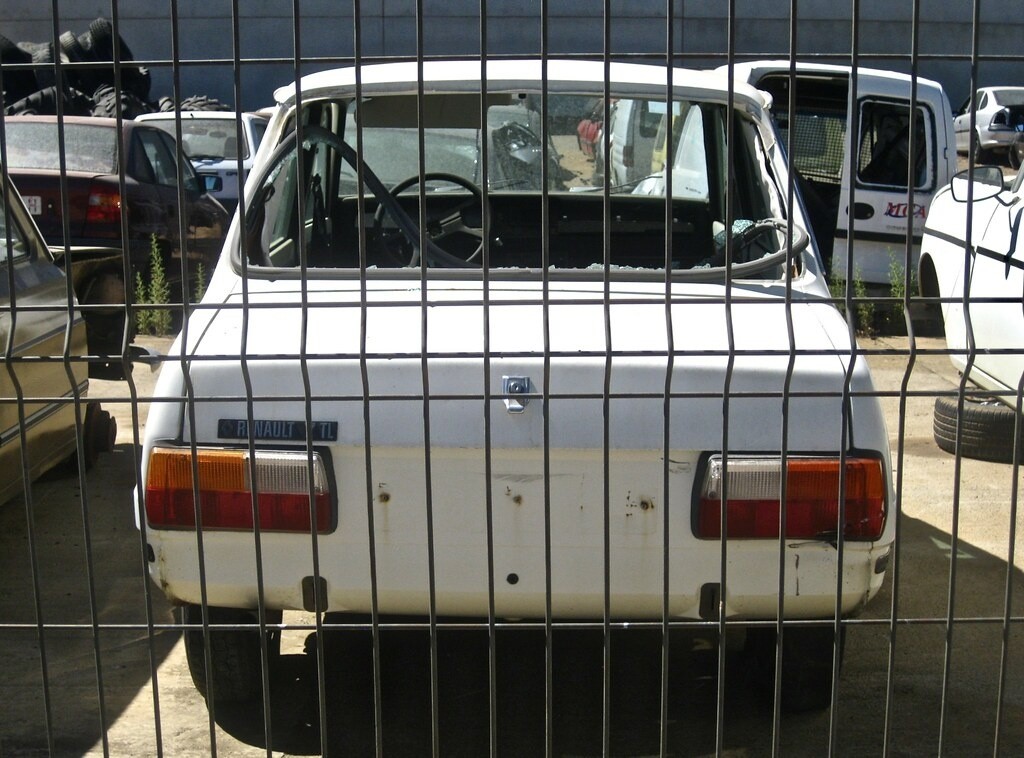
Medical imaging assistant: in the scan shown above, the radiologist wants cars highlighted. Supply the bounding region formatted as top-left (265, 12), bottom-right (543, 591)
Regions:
top-left (135, 54), bottom-right (898, 717)
top-left (595, 99), bottom-right (666, 193)
top-left (311, 116), bottom-right (563, 194)
top-left (3, 115), bottom-right (232, 300)
top-left (132, 110), bottom-right (270, 218)
top-left (576, 97), bottom-right (618, 159)
top-left (650, 102), bottom-right (846, 185)
top-left (954, 86), bottom-right (1024, 163)
top-left (919, 154), bottom-right (1023, 417)
top-left (487, 105), bottom-right (556, 159)
top-left (0, 168), bottom-right (116, 507)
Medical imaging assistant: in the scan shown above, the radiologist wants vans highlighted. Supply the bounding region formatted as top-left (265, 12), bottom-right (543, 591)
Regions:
top-left (631, 60), bottom-right (956, 334)
